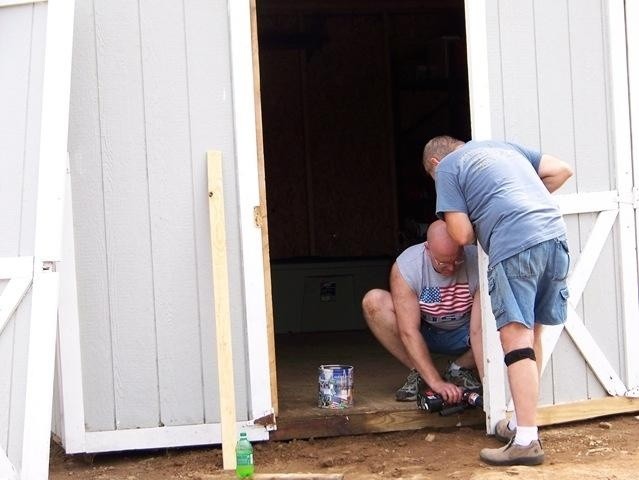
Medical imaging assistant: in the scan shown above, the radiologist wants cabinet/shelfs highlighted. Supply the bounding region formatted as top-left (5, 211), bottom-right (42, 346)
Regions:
top-left (235, 431), bottom-right (255, 478)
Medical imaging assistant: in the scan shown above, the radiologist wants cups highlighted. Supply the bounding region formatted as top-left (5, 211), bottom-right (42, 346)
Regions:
top-left (431, 252), bottom-right (465, 268)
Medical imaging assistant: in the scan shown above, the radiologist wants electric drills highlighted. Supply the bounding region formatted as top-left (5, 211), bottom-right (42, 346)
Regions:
top-left (417, 386), bottom-right (483, 416)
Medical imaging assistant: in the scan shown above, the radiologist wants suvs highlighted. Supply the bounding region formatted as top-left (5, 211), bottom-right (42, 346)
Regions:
top-left (318, 365), bottom-right (354, 408)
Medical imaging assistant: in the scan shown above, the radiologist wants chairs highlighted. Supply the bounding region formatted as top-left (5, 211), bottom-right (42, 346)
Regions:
top-left (394, 369), bottom-right (419, 401)
top-left (439, 360), bottom-right (482, 389)
top-left (494, 418), bottom-right (518, 442)
top-left (480, 435), bottom-right (545, 465)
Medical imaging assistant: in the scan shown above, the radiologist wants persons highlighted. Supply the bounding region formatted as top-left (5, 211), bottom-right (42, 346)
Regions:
top-left (362, 219), bottom-right (485, 406)
top-left (421, 134), bottom-right (577, 468)
